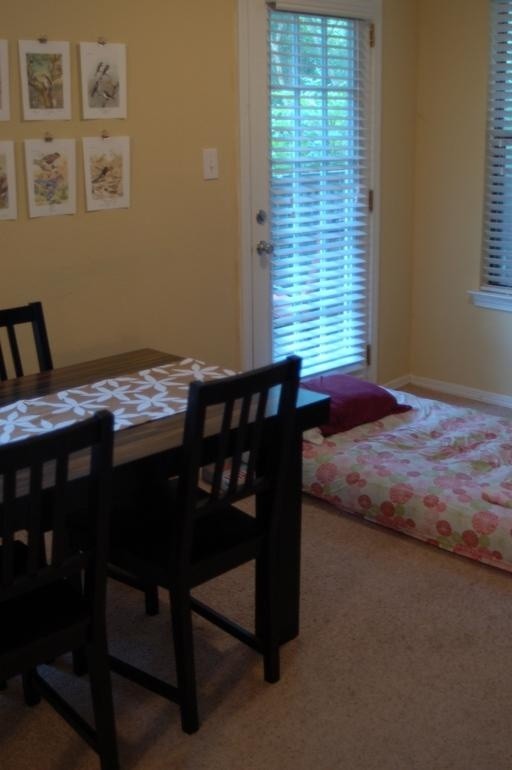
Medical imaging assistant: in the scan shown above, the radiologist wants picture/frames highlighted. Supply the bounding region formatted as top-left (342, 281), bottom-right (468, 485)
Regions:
top-left (0, 38), bottom-right (130, 221)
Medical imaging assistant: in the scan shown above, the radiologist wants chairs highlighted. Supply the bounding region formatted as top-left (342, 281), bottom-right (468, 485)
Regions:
top-left (0, 411), bottom-right (123, 768)
top-left (84, 354), bottom-right (301, 735)
top-left (0, 295), bottom-right (55, 381)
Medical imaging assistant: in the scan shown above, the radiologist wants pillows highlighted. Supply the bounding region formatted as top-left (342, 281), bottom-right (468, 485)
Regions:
top-left (297, 371), bottom-right (412, 446)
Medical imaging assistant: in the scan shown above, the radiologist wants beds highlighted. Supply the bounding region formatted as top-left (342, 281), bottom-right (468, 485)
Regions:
top-left (238, 376), bottom-right (512, 575)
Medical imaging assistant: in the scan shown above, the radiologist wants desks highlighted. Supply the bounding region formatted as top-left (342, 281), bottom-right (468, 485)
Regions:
top-left (1, 346), bottom-right (332, 642)
